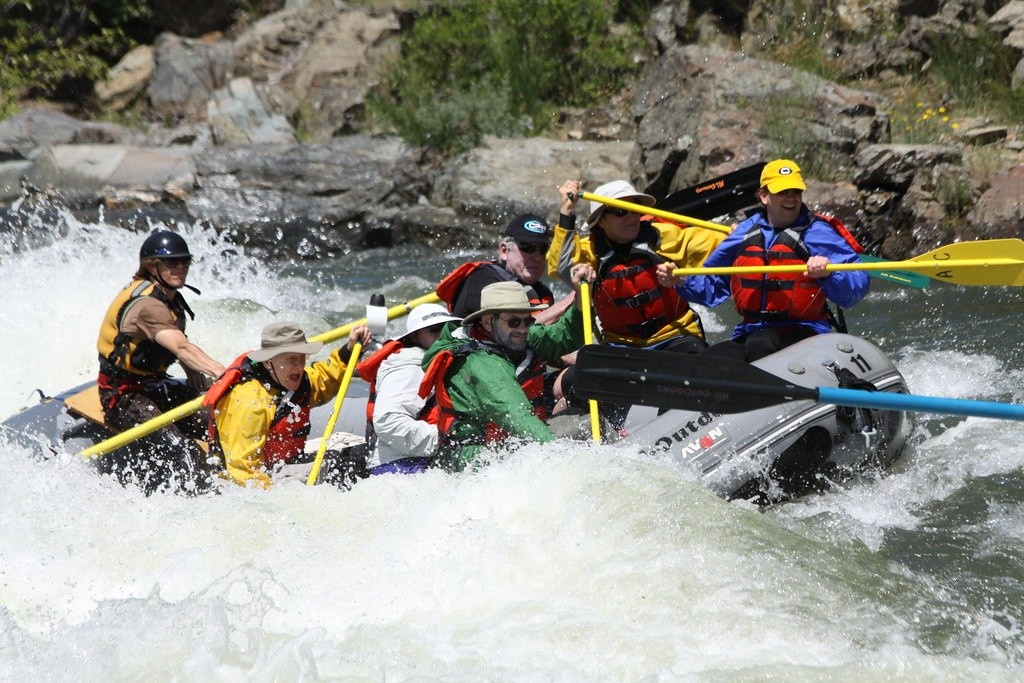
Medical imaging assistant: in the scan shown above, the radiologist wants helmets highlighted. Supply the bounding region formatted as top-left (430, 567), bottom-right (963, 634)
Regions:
top-left (139, 231), bottom-right (192, 259)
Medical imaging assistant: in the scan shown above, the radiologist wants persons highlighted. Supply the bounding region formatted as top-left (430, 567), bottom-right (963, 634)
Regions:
top-left (201, 321), bottom-right (372, 491)
top-left (357, 301), bottom-right (464, 475)
top-left (435, 214), bottom-right (579, 415)
top-left (421, 263), bottom-right (597, 470)
top-left (547, 179), bottom-right (739, 348)
top-left (657, 158), bottom-right (870, 341)
top-left (96, 232), bottom-right (227, 496)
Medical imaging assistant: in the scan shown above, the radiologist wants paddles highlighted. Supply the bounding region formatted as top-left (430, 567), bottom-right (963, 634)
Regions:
top-left (79, 290), bottom-right (445, 464)
top-left (671, 238), bottom-right (1024, 287)
top-left (564, 179), bottom-right (931, 287)
top-left (305, 336), bottom-right (362, 486)
top-left (572, 343), bottom-right (1024, 425)
top-left (581, 274), bottom-right (600, 441)
top-left (652, 161), bottom-right (775, 222)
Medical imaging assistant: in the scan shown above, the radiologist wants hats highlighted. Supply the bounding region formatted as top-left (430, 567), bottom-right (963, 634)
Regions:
top-left (504, 213), bottom-right (552, 244)
top-left (580, 180), bottom-right (656, 231)
top-left (247, 320), bottom-right (324, 363)
top-left (460, 280), bottom-right (549, 327)
top-left (760, 159), bottom-right (807, 194)
top-left (395, 303), bottom-right (467, 340)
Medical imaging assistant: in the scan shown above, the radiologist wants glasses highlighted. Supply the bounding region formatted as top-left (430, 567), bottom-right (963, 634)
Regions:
top-left (777, 189), bottom-right (802, 197)
top-left (494, 315), bottom-right (536, 328)
top-left (276, 353), bottom-right (309, 364)
top-left (510, 239), bottom-right (548, 255)
top-left (418, 326), bottom-right (442, 335)
top-left (605, 208), bottom-right (628, 217)
top-left (161, 260), bottom-right (190, 268)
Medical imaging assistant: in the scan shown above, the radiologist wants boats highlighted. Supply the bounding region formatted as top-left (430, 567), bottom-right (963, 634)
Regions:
top-left (0, 329), bottom-right (913, 527)
